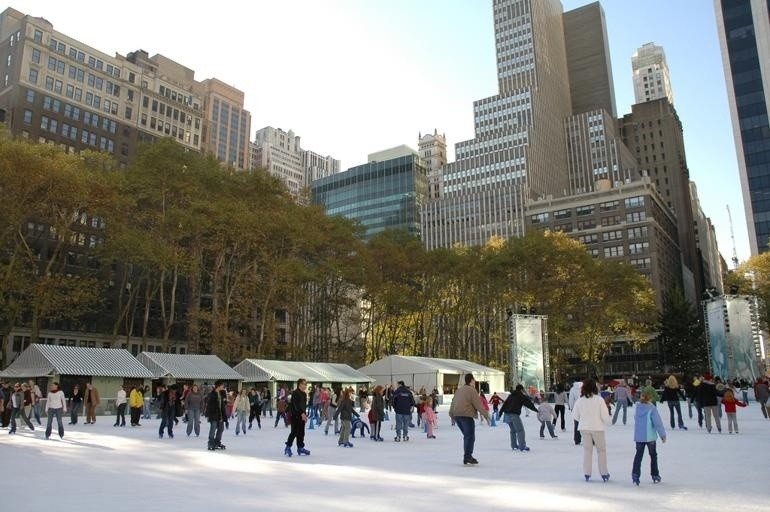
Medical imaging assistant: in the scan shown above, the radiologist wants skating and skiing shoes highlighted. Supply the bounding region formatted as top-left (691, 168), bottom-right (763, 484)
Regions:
top-left (248, 423), bottom-right (252, 429)
top-left (601, 473), bottom-right (610, 482)
top-left (73, 420), bottom-right (78, 424)
top-left (370, 436), bottom-right (375, 441)
top-left (344, 442), bottom-right (353, 448)
top-left (159, 431), bottom-right (164, 438)
top-left (623, 422), bottom-right (626, 424)
top-left (394, 437), bottom-right (400, 441)
top-left (464, 457), bottom-right (477, 464)
top-left (215, 440), bottom-right (226, 449)
top-left (84, 421), bottom-right (90, 424)
top-left (9, 429), bottom-right (15, 434)
top-left (137, 423), bottom-right (142, 426)
top-left (120, 420), bottom-right (126, 427)
top-left (652, 473), bottom-right (662, 484)
top-left (30, 427), bottom-right (35, 431)
top-left (375, 434), bottom-right (384, 441)
top-left (552, 436), bottom-right (558, 439)
top-left (718, 428), bottom-right (721, 433)
top-left (114, 420), bottom-right (120, 427)
top-left (361, 433), bottom-right (366, 437)
top-left (131, 423), bottom-right (136, 427)
top-left (91, 421), bottom-right (96, 425)
top-left (335, 431), bottom-right (340, 434)
top-left (236, 432), bottom-right (240, 435)
top-left (553, 424), bottom-right (555, 427)
top-left (46, 433), bottom-right (50, 439)
top-left (297, 448), bottom-right (310, 456)
top-left (338, 442), bottom-right (344, 446)
top-left (68, 421), bottom-right (73, 425)
top-left (585, 475), bottom-right (591, 482)
top-left (428, 435), bottom-right (435, 438)
top-left (325, 431), bottom-right (327, 435)
top-left (540, 436), bottom-right (544, 438)
top-left (520, 446), bottom-right (530, 452)
top-left (679, 424), bottom-right (687, 430)
top-left (707, 427), bottom-right (712, 433)
top-left (511, 446), bottom-right (518, 450)
top-left (764, 416), bottom-right (767, 418)
top-left (632, 474), bottom-right (640, 486)
top-left (403, 436), bottom-right (409, 441)
top-left (167, 431), bottom-right (174, 439)
top-left (208, 441), bottom-right (215, 450)
top-left (561, 428), bottom-right (566, 431)
top-left (285, 445), bottom-right (292, 457)
top-left (258, 424), bottom-right (261, 428)
top-left (612, 422), bottom-right (615, 425)
top-left (60, 432), bottom-right (64, 439)
top-left (243, 431), bottom-right (246, 434)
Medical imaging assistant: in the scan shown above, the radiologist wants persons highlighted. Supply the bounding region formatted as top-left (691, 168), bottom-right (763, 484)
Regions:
top-left (642, 379), bottom-right (662, 407)
top-left (685, 374), bottom-right (770, 433)
top-left (45, 382), bottom-right (66, 440)
top-left (488, 382), bottom-right (538, 451)
top-left (68, 386), bottom-right (84, 424)
top-left (573, 379), bottom-right (611, 482)
top-left (568, 376), bottom-right (586, 445)
top-left (448, 374), bottom-right (494, 464)
top-left (128, 373), bottom-right (439, 457)
top-left (84, 383), bottom-right (100, 424)
top-left (659, 376), bottom-right (687, 430)
top-left (537, 385), bottom-right (568, 439)
top-left (0, 380), bottom-right (43, 434)
top-left (601, 382), bottom-right (637, 424)
top-left (451, 419), bottom-right (456, 426)
top-left (632, 387), bottom-right (667, 485)
top-left (114, 385), bottom-right (127, 427)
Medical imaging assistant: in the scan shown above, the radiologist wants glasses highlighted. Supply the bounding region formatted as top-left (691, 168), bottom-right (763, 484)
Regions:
top-left (14, 386), bottom-right (19, 388)
top-left (352, 393), bottom-right (354, 394)
top-left (73, 387), bottom-right (79, 389)
